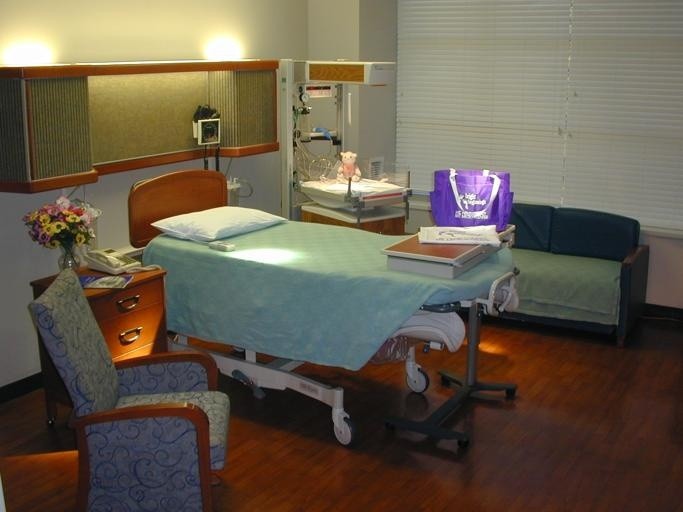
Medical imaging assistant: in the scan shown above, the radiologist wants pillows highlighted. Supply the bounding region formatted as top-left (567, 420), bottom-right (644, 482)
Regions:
top-left (150, 206), bottom-right (287, 242)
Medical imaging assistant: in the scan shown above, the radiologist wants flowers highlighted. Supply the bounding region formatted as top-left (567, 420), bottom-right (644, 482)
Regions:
top-left (22, 195), bottom-right (103, 249)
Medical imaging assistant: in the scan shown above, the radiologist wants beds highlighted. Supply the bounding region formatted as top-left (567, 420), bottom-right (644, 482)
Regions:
top-left (128, 169), bottom-right (519, 448)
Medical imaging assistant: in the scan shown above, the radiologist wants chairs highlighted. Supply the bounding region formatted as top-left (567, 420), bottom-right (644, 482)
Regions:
top-left (28, 268), bottom-right (232, 511)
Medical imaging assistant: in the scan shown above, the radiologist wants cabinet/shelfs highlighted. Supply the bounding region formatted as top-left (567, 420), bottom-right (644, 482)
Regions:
top-left (300, 211), bottom-right (405, 235)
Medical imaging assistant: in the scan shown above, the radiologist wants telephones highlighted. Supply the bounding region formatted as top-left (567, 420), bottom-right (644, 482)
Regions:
top-left (83, 248), bottom-right (141, 275)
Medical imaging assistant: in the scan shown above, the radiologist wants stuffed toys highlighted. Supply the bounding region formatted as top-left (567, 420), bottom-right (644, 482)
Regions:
top-left (336, 151), bottom-right (361, 181)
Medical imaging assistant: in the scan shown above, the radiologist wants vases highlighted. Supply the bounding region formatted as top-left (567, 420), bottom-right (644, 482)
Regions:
top-left (58, 253), bottom-right (81, 273)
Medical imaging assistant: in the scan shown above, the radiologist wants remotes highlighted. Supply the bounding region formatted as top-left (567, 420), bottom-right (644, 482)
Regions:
top-left (209, 241), bottom-right (235, 251)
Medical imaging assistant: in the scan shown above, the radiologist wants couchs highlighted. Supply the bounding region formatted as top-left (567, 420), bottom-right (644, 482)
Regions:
top-left (491, 202), bottom-right (649, 350)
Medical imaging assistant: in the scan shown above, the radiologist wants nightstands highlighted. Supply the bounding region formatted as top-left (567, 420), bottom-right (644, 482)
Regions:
top-left (30, 261), bottom-right (168, 423)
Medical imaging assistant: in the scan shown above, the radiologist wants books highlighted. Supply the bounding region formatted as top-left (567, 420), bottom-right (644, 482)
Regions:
top-left (78, 275), bottom-right (134, 289)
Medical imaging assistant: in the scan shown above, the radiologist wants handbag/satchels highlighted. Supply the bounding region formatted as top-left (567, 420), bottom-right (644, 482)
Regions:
top-left (429, 169), bottom-right (514, 233)
